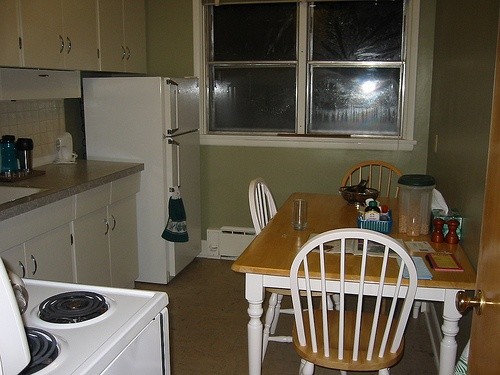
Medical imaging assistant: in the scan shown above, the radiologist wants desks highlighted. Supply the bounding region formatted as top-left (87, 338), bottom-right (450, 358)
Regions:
top-left (231, 192), bottom-right (478, 375)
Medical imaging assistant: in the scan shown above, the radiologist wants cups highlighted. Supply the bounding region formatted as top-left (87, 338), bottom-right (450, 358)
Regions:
top-left (407, 209), bottom-right (421, 236)
top-left (292, 199), bottom-right (307, 231)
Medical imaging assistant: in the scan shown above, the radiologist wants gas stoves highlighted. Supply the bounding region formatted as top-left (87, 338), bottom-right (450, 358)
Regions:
top-left (0, 256), bottom-right (169, 375)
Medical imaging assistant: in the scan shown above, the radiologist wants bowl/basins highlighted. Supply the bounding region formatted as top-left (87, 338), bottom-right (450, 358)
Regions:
top-left (339, 185), bottom-right (379, 204)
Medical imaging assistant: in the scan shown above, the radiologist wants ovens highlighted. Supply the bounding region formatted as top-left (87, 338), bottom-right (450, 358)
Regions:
top-left (99, 307), bottom-right (170, 375)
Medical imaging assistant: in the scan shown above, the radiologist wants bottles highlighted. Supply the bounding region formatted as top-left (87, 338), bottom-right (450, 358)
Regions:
top-left (365, 201), bottom-right (380, 220)
top-left (0, 135), bottom-right (17, 183)
top-left (15, 137), bottom-right (34, 177)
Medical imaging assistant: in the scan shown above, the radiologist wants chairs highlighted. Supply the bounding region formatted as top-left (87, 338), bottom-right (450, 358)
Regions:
top-left (248, 161), bottom-right (422, 375)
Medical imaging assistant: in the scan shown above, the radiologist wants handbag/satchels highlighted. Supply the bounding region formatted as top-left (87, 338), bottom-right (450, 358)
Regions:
top-left (158, 187), bottom-right (190, 243)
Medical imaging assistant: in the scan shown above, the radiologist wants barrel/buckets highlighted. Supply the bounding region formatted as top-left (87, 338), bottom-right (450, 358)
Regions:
top-left (398, 174), bottom-right (436, 235)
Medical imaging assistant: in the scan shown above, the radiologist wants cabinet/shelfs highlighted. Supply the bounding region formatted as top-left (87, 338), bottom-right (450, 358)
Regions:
top-left (0, 171), bottom-right (141, 289)
top-left (0, 0), bottom-right (193, 78)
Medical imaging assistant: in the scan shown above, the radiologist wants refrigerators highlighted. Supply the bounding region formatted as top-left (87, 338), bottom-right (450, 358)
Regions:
top-left (82, 77), bottom-right (201, 284)
